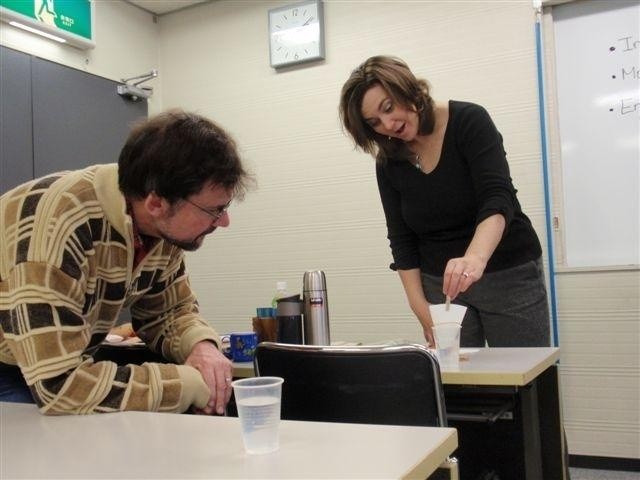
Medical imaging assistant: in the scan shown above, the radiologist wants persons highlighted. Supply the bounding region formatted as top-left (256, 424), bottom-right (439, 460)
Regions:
top-left (1, 111), bottom-right (257, 415)
top-left (340, 55), bottom-right (550, 348)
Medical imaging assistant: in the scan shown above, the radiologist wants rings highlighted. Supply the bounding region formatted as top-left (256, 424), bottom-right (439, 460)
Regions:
top-left (463, 272), bottom-right (468, 277)
top-left (226, 378), bottom-right (232, 381)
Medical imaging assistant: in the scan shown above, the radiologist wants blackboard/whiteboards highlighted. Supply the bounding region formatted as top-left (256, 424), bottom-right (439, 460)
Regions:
top-left (544, 0), bottom-right (640, 274)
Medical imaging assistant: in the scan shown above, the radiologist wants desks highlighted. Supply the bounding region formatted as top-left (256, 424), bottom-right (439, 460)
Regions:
top-left (1, 398), bottom-right (460, 480)
top-left (231, 342), bottom-right (560, 480)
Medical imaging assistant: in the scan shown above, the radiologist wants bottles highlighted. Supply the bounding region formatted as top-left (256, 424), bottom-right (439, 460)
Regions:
top-left (303, 269), bottom-right (332, 346)
top-left (271, 281), bottom-right (288, 308)
top-left (251, 295), bottom-right (303, 344)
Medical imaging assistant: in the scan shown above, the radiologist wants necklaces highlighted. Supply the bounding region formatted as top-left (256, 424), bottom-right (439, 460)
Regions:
top-left (414, 150), bottom-right (421, 169)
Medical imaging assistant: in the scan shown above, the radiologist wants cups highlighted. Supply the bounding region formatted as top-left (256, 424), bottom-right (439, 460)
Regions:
top-left (220, 331), bottom-right (257, 362)
top-left (432, 323), bottom-right (460, 371)
top-left (230, 376), bottom-right (284, 456)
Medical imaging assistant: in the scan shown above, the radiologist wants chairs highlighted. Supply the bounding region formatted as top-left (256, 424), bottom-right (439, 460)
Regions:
top-left (254, 341), bottom-right (450, 427)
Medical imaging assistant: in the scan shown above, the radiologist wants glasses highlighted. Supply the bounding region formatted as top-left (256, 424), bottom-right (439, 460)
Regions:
top-left (178, 199), bottom-right (234, 223)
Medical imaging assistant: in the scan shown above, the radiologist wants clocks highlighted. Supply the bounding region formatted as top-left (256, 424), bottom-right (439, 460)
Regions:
top-left (267, 0), bottom-right (324, 68)
